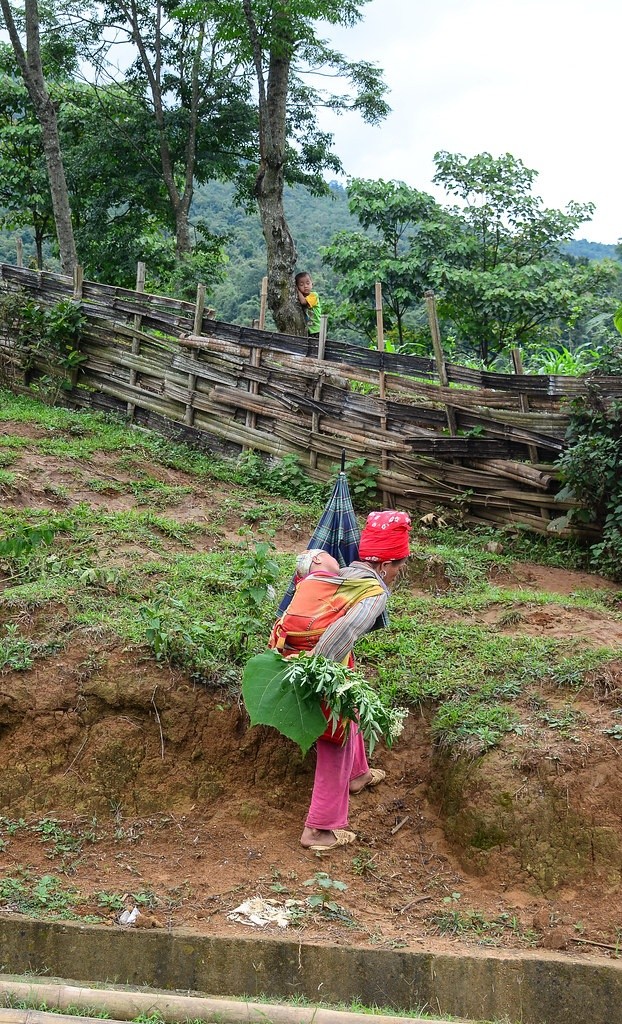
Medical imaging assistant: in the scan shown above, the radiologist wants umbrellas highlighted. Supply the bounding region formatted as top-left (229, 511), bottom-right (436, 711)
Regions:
top-left (275, 470), bottom-right (391, 640)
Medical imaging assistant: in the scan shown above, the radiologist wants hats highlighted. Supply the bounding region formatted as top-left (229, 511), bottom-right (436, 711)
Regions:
top-left (296, 549), bottom-right (327, 578)
top-left (357, 511), bottom-right (413, 563)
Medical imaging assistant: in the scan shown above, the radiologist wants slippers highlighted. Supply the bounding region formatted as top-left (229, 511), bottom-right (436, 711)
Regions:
top-left (310, 829), bottom-right (356, 851)
top-left (352, 768), bottom-right (386, 794)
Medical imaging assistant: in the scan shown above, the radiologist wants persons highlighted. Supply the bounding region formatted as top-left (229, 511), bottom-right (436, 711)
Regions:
top-left (277, 548), bottom-right (351, 673)
top-left (268, 507), bottom-right (413, 852)
top-left (295, 271), bottom-right (324, 338)
